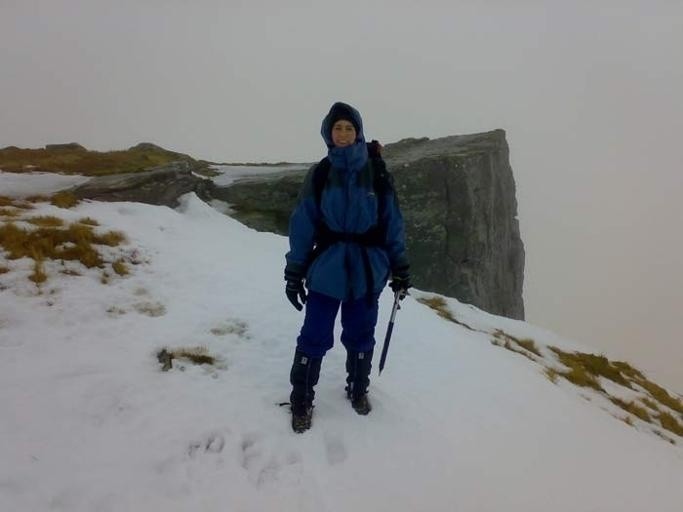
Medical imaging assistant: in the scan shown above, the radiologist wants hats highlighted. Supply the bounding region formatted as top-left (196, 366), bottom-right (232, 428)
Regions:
top-left (329, 102), bottom-right (360, 135)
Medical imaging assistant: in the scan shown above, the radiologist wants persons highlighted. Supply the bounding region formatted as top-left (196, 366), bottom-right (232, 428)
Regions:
top-left (283, 102), bottom-right (412, 434)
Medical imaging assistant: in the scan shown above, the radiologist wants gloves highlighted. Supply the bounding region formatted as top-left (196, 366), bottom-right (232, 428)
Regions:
top-left (391, 270), bottom-right (410, 300)
top-left (285, 264), bottom-right (306, 310)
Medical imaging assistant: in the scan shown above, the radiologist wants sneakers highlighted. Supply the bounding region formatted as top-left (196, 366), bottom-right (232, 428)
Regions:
top-left (347, 391), bottom-right (372, 415)
top-left (290, 398), bottom-right (313, 432)
